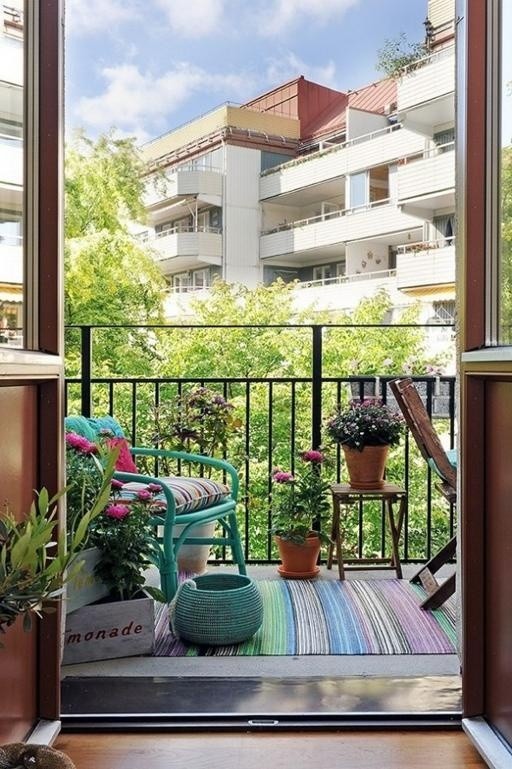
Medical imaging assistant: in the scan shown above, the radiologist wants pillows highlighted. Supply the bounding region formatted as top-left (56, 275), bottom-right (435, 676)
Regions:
top-left (109, 477), bottom-right (233, 515)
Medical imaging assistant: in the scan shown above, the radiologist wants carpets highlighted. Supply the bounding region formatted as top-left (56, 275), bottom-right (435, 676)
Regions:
top-left (140, 568), bottom-right (457, 657)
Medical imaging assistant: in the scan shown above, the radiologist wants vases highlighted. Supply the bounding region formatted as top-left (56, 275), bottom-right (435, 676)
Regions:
top-left (275, 530), bottom-right (321, 572)
top-left (60, 530), bottom-right (155, 667)
top-left (173, 520), bottom-right (216, 575)
top-left (342, 443), bottom-right (389, 482)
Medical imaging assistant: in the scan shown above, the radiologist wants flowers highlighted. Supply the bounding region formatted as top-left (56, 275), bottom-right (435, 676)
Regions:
top-left (326, 399), bottom-right (405, 452)
top-left (265, 451), bottom-right (335, 547)
top-left (66, 426), bottom-right (164, 601)
top-left (150, 387), bottom-right (241, 479)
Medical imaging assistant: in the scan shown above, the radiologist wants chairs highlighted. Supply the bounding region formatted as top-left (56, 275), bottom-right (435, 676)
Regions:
top-left (65, 414), bottom-right (247, 607)
top-left (388, 378), bottom-right (457, 609)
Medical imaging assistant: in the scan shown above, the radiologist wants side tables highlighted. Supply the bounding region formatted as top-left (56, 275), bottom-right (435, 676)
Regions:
top-left (327, 483), bottom-right (407, 580)
